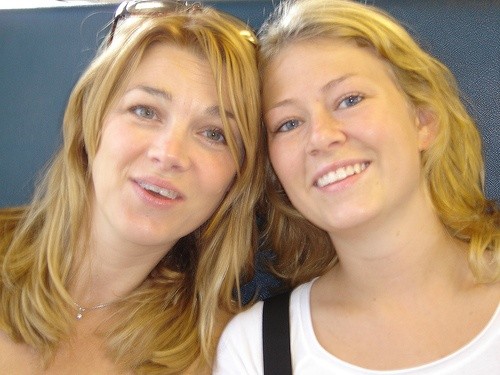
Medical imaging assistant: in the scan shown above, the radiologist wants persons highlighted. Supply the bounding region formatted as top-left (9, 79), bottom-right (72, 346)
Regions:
top-left (207, 0), bottom-right (500, 375)
top-left (0, 1), bottom-right (273, 374)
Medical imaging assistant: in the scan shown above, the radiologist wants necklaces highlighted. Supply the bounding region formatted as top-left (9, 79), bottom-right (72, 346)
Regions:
top-left (50, 215), bottom-right (151, 320)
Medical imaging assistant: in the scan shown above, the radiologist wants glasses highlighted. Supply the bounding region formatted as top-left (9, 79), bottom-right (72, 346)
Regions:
top-left (111, 1), bottom-right (261, 66)
